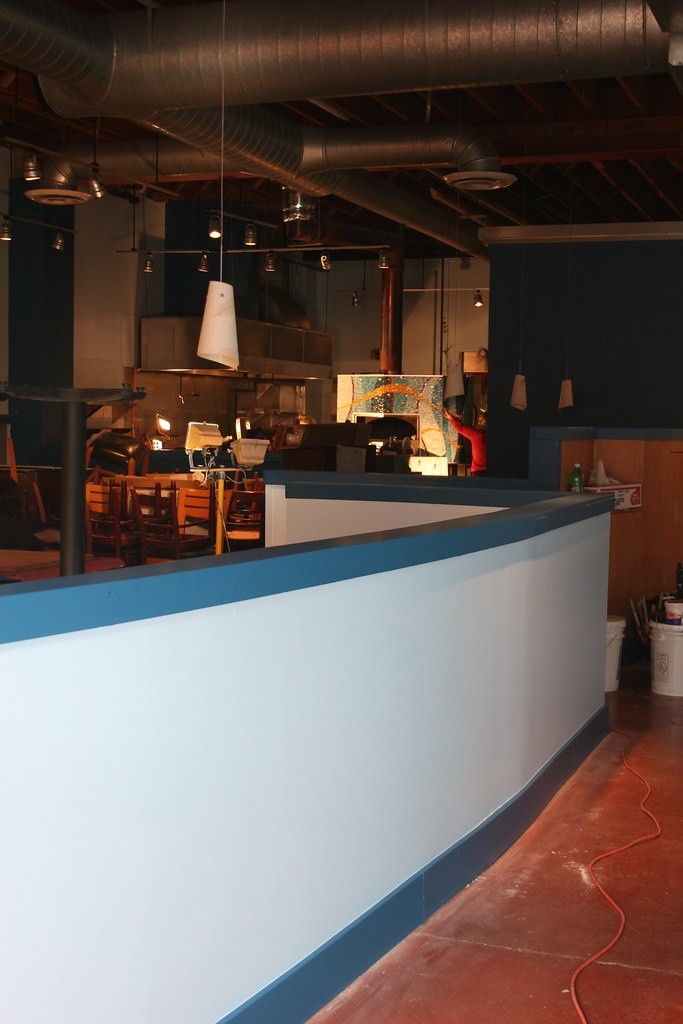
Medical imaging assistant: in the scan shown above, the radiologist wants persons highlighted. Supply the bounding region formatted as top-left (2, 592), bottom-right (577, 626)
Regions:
top-left (443, 407), bottom-right (487, 477)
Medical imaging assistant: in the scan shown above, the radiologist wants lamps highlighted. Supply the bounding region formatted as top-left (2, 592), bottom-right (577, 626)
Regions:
top-left (197, 0), bottom-right (240, 371)
top-left (144, 252), bottom-right (154, 273)
top-left (321, 249), bottom-right (332, 271)
top-left (155, 413), bottom-right (171, 441)
top-left (0, 216), bottom-right (12, 241)
top-left (265, 249), bottom-right (278, 271)
top-left (510, 83), bottom-right (529, 411)
top-left (209, 212), bottom-right (221, 239)
top-left (377, 248), bottom-right (389, 269)
top-left (353, 291), bottom-right (359, 308)
top-left (185, 421), bottom-right (222, 470)
top-left (443, 86), bottom-right (463, 399)
top-left (52, 229), bottom-right (64, 255)
top-left (198, 251), bottom-right (209, 272)
top-left (230, 417), bottom-right (270, 467)
top-left (558, 126), bottom-right (574, 411)
top-left (244, 220), bottom-right (257, 245)
top-left (88, 163), bottom-right (106, 198)
top-left (474, 290), bottom-right (483, 307)
top-left (23, 147), bottom-right (41, 181)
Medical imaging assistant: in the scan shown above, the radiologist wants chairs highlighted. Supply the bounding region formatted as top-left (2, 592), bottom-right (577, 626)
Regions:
top-left (85, 473), bottom-right (265, 574)
top-left (12, 472), bottom-right (61, 545)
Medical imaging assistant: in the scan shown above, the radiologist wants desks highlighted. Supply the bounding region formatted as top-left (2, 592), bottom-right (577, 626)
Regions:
top-left (0, 549), bottom-right (61, 582)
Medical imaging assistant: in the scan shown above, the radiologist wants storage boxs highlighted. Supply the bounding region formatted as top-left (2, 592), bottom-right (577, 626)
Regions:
top-left (583, 482), bottom-right (642, 511)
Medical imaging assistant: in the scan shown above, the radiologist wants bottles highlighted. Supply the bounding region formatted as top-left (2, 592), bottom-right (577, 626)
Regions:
top-left (570, 464), bottom-right (583, 493)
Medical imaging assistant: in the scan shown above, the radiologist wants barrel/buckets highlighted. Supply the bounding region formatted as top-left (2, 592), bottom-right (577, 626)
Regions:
top-left (648, 619), bottom-right (683, 696)
top-left (605, 615), bottom-right (627, 692)
top-left (665, 601), bottom-right (683, 620)
